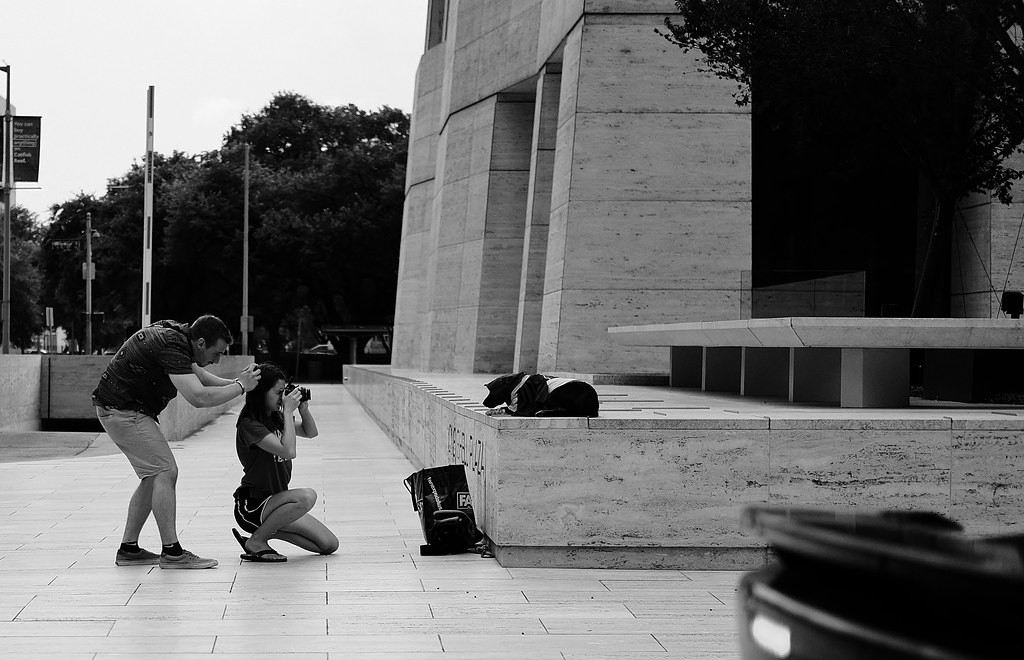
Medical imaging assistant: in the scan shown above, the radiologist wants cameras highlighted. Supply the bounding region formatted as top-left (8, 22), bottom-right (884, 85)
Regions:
top-left (253, 364), bottom-right (258, 372)
top-left (284, 383), bottom-right (311, 402)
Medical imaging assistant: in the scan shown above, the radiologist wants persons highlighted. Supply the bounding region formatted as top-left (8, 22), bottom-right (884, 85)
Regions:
top-left (92, 315), bottom-right (261, 568)
top-left (232, 363), bottom-right (339, 562)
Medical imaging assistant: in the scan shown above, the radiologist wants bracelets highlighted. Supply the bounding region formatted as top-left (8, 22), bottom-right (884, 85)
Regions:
top-left (236, 379), bottom-right (245, 395)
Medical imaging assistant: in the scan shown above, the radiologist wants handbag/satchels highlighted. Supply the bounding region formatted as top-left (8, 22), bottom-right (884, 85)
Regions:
top-left (404, 464), bottom-right (484, 552)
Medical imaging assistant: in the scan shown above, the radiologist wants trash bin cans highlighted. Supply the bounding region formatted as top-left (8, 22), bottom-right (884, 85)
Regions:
top-left (737, 507), bottom-right (1024, 660)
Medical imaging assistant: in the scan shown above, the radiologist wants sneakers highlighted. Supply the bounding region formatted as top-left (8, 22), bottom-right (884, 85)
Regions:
top-left (159, 550), bottom-right (218, 569)
top-left (115, 547), bottom-right (162, 565)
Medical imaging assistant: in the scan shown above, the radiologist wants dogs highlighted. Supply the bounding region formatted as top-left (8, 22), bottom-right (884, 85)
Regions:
top-left (482, 372), bottom-right (600, 417)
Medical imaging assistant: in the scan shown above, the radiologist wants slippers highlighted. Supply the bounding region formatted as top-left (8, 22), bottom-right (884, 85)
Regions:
top-left (240, 550), bottom-right (288, 562)
top-left (232, 527), bottom-right (250, 553)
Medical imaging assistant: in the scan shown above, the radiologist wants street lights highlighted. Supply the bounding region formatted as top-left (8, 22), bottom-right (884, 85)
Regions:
top-left (85, 212), bottom-right (100, 353)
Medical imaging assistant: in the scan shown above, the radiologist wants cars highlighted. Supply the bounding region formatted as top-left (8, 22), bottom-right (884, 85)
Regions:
top-left (302, 343), bottom-right (339, 355)
top-left (364, 340), bottom-right (391, 355)
